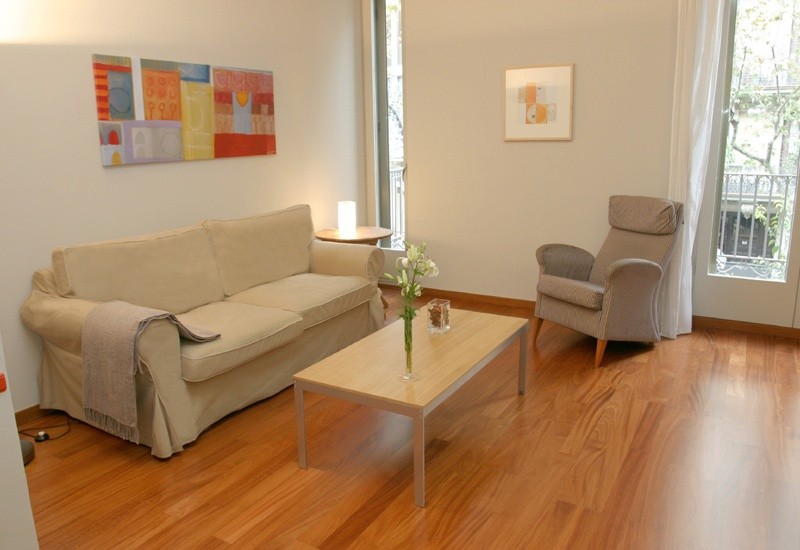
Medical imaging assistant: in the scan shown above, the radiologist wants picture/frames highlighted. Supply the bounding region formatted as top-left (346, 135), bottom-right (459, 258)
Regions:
top-left (502, 63), bottom-right (574, 144)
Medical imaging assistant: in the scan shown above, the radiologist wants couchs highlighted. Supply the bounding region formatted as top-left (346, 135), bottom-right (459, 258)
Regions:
top-left (20, 204), bottom-right (385, 459)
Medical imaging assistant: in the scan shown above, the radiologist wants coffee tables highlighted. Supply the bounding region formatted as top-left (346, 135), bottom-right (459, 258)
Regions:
top-left (292, 304), bottom-right (528, 508)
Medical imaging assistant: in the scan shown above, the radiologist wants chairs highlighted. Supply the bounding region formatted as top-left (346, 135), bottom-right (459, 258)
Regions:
top-left (531, 195), bottom-right (685, 370)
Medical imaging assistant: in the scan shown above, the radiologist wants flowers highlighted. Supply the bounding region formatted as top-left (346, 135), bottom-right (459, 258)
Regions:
top-left (383, 239), bottom-right (440, 378)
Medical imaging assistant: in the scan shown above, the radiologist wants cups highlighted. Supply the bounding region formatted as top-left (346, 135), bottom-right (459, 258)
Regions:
top-left (426, 298), bottom-right (451, 333)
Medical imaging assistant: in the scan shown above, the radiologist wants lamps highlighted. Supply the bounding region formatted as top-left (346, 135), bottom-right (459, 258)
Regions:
top-left (338, 199), bottom-right (357, 235)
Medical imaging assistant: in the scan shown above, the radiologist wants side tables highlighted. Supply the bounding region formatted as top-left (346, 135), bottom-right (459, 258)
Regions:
top-left (315, 225), bottom-right (393, 308)
